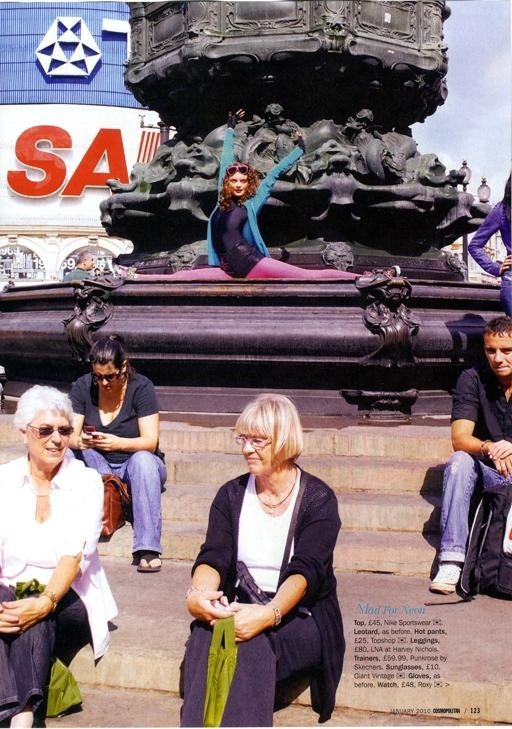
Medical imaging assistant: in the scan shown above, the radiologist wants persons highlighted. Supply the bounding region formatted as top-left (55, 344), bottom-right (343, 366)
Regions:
top-left (466, 168), bottom-right (511, 318)
top-left (62, 251), bottom-right (96, 279)
top-left (62, 337), bottom-right (169, 571)
top-left (178, 388), bottom-right (347, 727)
top-left (0, 385), bottom-right (110, 728)
top-left (116, 107), bottom-right (401, 282)
top-left (427, 317), bottom-right (511, 593)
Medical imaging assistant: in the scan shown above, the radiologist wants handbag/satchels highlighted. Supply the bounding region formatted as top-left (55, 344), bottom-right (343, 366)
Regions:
top-left (233, 560), bottom-right (272, 607)
top-left (100, 473), bottom-right (132, 537)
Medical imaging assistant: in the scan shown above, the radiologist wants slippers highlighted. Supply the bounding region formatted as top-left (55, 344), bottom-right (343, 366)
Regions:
top-left (135, 551), bottom-right (165, 573)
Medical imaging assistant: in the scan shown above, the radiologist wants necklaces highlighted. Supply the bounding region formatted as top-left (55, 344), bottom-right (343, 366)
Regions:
top-left (29, 461), bottom-right (62, 496)
top-left (254, 466), bottom-right (299, 514)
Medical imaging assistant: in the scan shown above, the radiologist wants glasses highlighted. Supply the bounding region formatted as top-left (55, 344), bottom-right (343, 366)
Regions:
top-left (26, 423), bottom-right (75, 438)
top-left (233, 435), bottom-right (271, 451)
top-left (89, 370), bottom-right (127, 382)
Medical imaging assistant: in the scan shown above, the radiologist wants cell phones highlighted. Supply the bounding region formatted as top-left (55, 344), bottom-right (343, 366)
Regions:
top-left (83, 425), bottom-right (97, 441)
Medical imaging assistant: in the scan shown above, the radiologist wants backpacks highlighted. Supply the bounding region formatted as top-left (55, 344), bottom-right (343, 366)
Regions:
top-left (454, 480), bottom-right (511, 602)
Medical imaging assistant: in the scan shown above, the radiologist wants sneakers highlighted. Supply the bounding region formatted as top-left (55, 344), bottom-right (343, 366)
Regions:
top-left (428, 578), bottom-right (457, 595)
top-left (383, 264), bottom-right (405, 278)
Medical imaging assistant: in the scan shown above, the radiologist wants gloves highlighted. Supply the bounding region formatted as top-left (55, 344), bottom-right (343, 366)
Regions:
top-left (224, 112), bottom-right (241, 129)
top-left (293, 133), bottom-right (307, 153)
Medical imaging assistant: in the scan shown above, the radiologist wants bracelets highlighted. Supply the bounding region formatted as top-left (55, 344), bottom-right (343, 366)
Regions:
top-left (40, 590), bottom-right (59, 614)
top-left (266, 601), bottom-right (282, 628)
top-left (479, 439), bottom-right (491, 462)
top-left (182, 586), bottom-right (201, 597)
